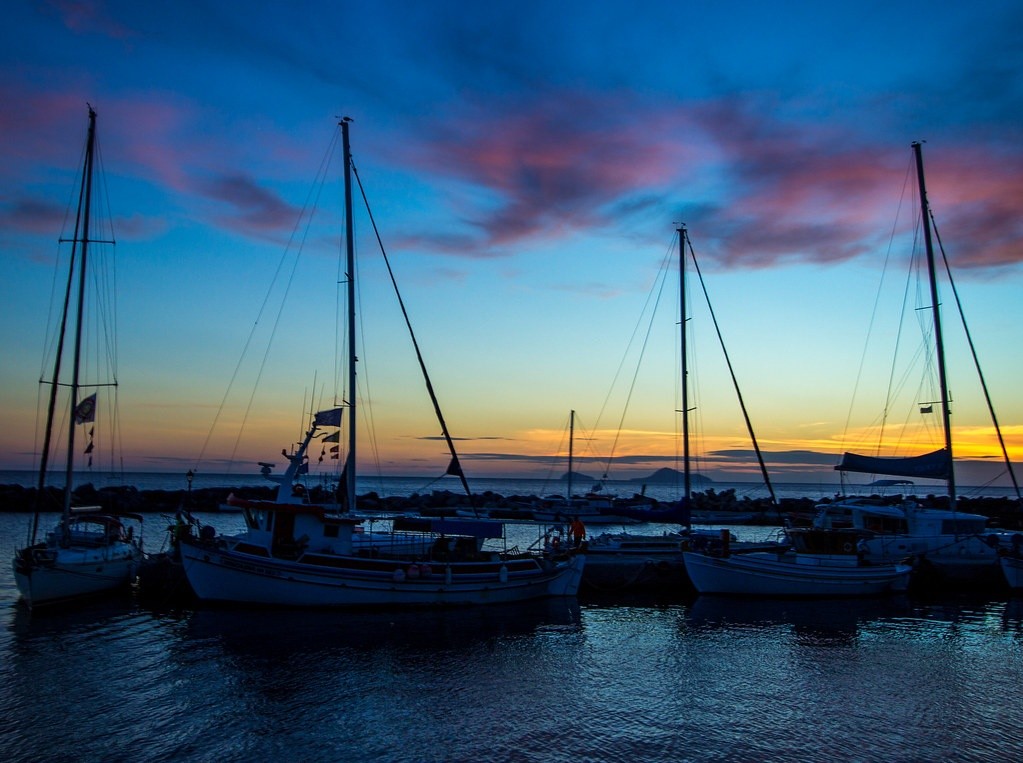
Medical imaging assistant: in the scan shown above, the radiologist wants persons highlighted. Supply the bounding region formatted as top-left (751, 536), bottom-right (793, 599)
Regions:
top-left (549, 525), bottom-right (564, 550)
top-left (567, 514), bottom-right (585, 550)
top-left (296, 536), bottom-right (309, 553)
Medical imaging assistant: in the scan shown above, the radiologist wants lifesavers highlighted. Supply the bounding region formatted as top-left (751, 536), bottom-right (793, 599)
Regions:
top-left (843, 542), bottom-right (854, 553)
top-left (553, 537), bottom-right (560, 548)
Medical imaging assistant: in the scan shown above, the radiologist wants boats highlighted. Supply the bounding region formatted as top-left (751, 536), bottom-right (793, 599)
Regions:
top-left (679, 549), bottom-right (912, 599)
top-left (689, 513), bottom-right (753, 524)
top-left (686, 595), bottom-right (884, 646)
top-left (997, 554), bottom-right (1023, 590)
top-left (813, 448), bottom-right (989, 533)
top-left (856, 528), bottom-right (1023, 572)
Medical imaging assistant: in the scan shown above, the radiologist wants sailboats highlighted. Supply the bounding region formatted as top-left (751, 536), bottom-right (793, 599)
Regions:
top-left (777, 140), bottom-right (1022, 556)
top-left (11, 105), bottom-right (142, 612)
top-left (187, 611), bottom-right (581, 656)
top-left (171, 117), bottom-right (590, 611)
top-left (527, 410), bottom-right (654, 524)
top-left (533, 218), bottom-right (804, 608)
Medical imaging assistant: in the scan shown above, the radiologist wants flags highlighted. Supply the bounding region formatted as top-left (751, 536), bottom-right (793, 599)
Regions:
top-left (88, 456), bottom-right (92, 467)
top-left (322, 431), bottom-right (340, 443)
top-left (84, 442), bottom-right (94, 454)
top-left (331, 454), bottom-right (339, 459)
top-left (89, 427), bottom-right (93, 436)
top-left (76, 393), bottom-right (96, 424)
top-left (330, 445), bottom-right (339, 452)
top-left (314, 408), bottom-right (343, 427)
